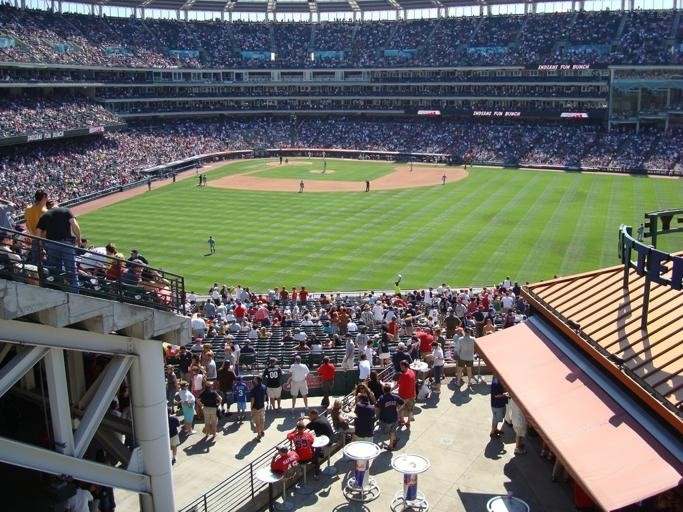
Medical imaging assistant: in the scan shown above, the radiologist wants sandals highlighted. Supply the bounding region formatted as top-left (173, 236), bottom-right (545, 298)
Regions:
top-left (514, 448), bottom-right (528, 454)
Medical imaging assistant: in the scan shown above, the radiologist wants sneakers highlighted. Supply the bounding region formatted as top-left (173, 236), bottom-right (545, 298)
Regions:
top-left (383, 421), bottom-right (410, 450)
top-left (183, 428), bottom-right (216, 444)
top-left (274, 406), bottom-right (309, 416)
top-left (490, 432), bottom-right (500, 439)
top-left (221, 409), bottom-right (245, 421)
top-left (495, 429), bottom-right (504, 435)
top-left (453, 380), bottom-right (461, 387)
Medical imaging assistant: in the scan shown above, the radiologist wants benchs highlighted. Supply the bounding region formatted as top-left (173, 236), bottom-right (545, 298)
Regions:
top-left (164, 296), bottom-right (504, 376)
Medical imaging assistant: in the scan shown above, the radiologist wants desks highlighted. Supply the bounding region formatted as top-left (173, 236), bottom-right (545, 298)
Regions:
top-left (410, 362), bottom-right (428, 399)
top-left (486, 494), bottom-right (530, 512)
top-left (256, 467), bottom-right (284, 512)
top-left (390, 454), bottom-right (430, 512)
top-left (311, 434), bottom-right (330, 481)
top-left (343, 441), bottom-right (380, 502)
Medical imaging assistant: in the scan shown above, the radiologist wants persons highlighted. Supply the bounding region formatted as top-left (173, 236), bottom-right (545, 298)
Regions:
top-left (1, 4), bottom-right (683, 481)
top-left (64, 480), bottom-right (94, 511)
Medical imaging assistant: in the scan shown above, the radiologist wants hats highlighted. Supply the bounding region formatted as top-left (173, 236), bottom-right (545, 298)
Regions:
top-left (131, 258), bottom-right (147, 266)
top-left (275, 447), bottom-right (288, 453)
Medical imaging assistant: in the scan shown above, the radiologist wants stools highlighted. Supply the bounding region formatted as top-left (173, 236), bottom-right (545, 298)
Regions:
top-left (321, 443), bottom-right (338, 475)
top-left (276, 473), bottom-right (296, 510)
top-left (295, 461), bottom-right (314, 495)
